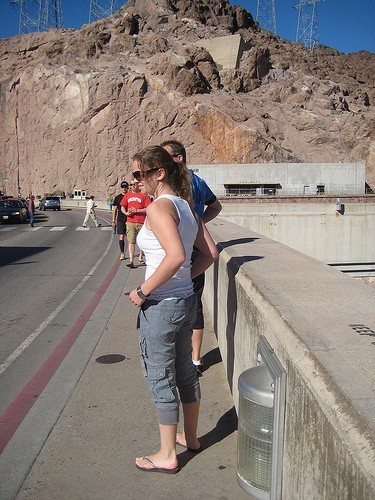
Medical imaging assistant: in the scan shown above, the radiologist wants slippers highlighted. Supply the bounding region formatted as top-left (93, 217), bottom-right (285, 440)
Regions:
top-left (138, 260), bottom-right (146, 266)
top-left (175, 432), bottom-right (203, 453)
top-left (136, 455), bottom-right (180, 473)
top-left (126, 262), bottom-right (134, 268)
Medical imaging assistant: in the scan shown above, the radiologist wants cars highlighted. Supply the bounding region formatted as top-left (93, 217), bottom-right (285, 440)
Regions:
top-left (0, 191), bottom-right (29, 224)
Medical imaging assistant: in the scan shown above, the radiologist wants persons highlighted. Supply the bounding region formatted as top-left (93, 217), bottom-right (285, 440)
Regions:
top-left (112, 177), bottom-right (152, 267)
top-left (83, 196), bottom-right (102, 228)
top-left (161, 140), bottom-right (222, 372)
top-left (128, 146), bottom-right (219, 474)
top-left (29, 194), bottom-right (35, 227)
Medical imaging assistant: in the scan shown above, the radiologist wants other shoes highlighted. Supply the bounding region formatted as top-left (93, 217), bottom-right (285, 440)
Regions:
top-left (193, 364), bottom-right (204, 376)
top-left (120, 253), bottom-right (125, 260)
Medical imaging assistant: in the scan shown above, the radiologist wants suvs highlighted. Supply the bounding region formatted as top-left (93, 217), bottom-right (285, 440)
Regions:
top-left (39, 196), bottom-right (61, 211)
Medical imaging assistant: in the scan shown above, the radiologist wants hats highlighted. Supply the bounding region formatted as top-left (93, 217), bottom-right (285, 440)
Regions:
top-left (30, 195), bottom-right (35, 199)
top-left (120, 182), bottom-right (128, 188)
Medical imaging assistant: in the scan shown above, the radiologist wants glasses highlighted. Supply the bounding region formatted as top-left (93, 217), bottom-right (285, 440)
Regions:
top-left (132, 166), bottom-right (164, 181)
top-left (130, 184), bottom-right (135, 186)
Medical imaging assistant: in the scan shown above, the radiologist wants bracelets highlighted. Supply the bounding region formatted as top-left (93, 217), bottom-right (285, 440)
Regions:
top-left (136, 209), bottom-right (137, 213)
top-left (113, 222), bottom-right (115, 223)
top-left (137, 286), bottom-right (151, 300)
